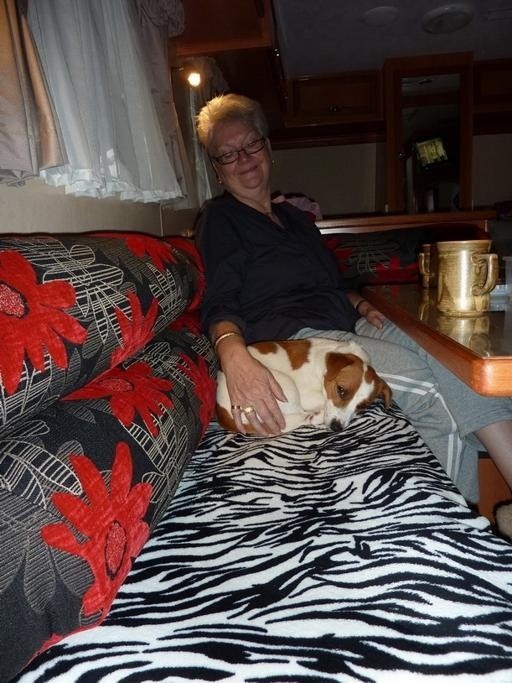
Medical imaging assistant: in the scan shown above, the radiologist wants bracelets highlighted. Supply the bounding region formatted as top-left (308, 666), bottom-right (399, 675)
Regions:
top-left (356, 299), bottom-right (366, 313)
top-left (213, 331), bottom-right (244, 353)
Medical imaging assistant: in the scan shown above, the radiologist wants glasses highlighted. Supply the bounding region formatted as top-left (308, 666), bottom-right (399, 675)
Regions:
top-left (209, 136), bottom-right (267, 165)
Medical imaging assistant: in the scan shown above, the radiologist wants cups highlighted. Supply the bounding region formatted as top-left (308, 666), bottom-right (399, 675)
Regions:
top-left (501, 254), bottom-right (511, 312)
top-left (436, 239), bottom-right (498, 319)
top-left (417, 242), bottom-right (436, 290)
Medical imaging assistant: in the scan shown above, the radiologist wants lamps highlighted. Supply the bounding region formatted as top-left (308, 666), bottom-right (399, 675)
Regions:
top-left (420, 4), bottom-right (476, 35)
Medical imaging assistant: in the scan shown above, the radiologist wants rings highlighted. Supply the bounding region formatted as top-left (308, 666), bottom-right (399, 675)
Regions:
top-left (244, 407), bottom-right (254, 415)
top-left (231, 406), bottom-right (242, 410)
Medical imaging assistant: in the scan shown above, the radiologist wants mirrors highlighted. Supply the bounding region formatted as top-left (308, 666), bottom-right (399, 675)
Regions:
top-left (392, 64), bottom-right (466, 213)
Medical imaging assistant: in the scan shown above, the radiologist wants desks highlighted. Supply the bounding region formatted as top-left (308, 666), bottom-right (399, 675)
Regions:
top-left (358, 283), bottom-right (512, 399)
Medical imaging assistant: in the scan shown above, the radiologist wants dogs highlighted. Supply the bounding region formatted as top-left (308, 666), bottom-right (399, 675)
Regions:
top-left (216, 336), bottom-right (392, 437)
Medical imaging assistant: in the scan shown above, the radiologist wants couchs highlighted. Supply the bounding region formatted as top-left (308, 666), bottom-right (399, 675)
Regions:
top-left (0, 221), bottom-right (512, 683)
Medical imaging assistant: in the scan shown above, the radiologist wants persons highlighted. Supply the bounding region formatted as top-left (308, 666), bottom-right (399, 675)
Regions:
top-left (194, 93), bottom-right (512, 544)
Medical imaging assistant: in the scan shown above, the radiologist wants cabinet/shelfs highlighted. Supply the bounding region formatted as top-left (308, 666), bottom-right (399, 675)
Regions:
top-left (170, 2), bottom-right (289, 142)
top-left (287, 70), bottom-right (385, 137)
top-left (473, 57), bottom-right (511, 136)
